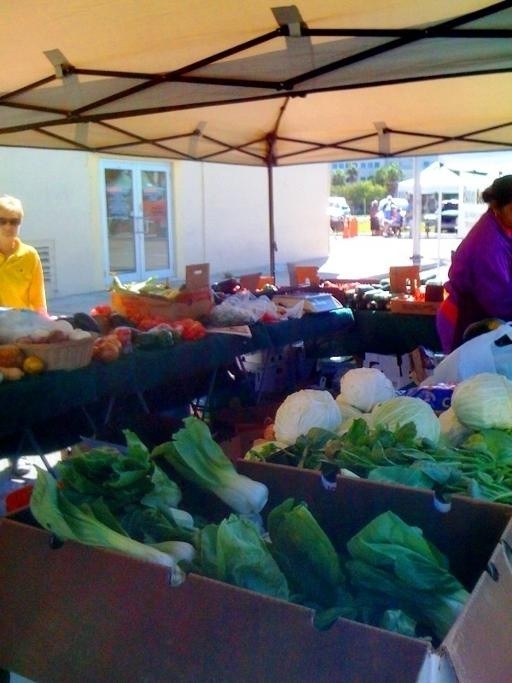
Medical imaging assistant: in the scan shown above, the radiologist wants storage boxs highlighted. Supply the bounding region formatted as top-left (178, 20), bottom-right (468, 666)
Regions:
top-left (0, 449), bottom-right (509, 682)
top-left (110, 260), bottom-right (213, 327)
top-left (363, 348), bottom-right (417, 394)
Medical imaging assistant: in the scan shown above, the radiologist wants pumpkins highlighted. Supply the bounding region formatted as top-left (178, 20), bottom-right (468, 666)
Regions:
top-left (0, 344), bottom-right (25, 368)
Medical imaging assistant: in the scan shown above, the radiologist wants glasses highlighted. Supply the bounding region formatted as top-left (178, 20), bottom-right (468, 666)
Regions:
top-left (1, 216), bottom-right (21, 225)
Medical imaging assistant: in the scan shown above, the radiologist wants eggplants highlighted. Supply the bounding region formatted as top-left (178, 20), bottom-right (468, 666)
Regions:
top-left (355, 299), bottom-right (381, 310)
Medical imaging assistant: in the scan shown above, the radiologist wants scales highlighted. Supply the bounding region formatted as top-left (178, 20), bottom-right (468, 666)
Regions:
top-left (272, 293), bottom-right (344, 313)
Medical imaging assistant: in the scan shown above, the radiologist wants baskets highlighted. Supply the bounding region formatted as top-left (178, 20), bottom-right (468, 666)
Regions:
top-left (13, 330), bottom-right (98, 372)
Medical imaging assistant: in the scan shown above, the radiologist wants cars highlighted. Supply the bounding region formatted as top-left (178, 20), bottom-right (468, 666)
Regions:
top-left (379, 196), bottom-right (411, 225)
top-left (330, 194), bottom-right (350, 228)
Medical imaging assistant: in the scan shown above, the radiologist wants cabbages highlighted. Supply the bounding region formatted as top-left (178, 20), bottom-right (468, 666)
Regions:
top-left (246, 365), bottom-right (511, 466)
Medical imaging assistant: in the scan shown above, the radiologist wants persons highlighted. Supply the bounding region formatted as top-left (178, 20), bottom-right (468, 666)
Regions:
top-left (382, 207), bottom-right (401, 237)
top-left (404, 196), bottom-right (413, 239)
top-left (432, 175), bottom-right (511, 359)
top-left (383, 193), bottom-right (396, 236)
top-left (370, 198), bottom-right (381, 236)
top-left (1, 193), bottom-right (48, 479)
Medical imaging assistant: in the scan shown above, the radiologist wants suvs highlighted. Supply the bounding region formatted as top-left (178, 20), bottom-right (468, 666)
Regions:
top-left (433, 197), bottom-right (459, 233)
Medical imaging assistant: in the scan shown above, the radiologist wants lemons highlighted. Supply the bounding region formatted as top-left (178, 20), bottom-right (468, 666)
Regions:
top-left (23, 356), bottom-right (44, 375)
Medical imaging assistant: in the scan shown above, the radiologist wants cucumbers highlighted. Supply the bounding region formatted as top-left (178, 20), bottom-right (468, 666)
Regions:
top-left (343, 280), bottom-right (390, 302)
